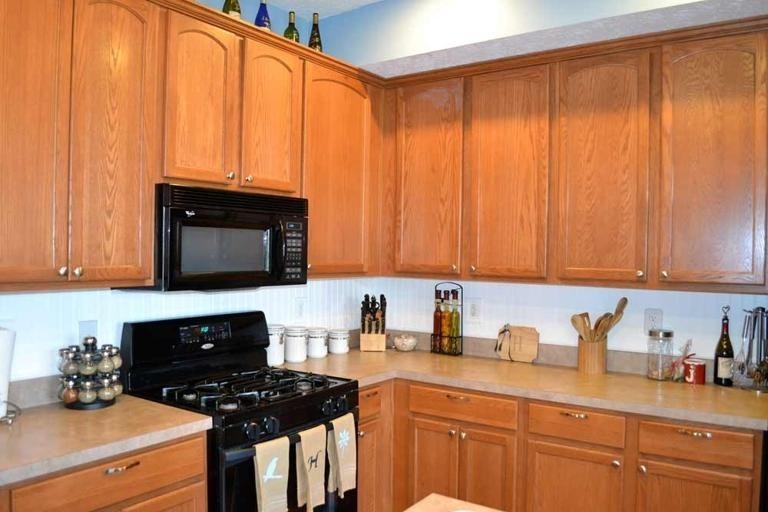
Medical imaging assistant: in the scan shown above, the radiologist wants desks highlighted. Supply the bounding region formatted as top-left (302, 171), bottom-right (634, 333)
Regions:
top-left (402, 487), bottom-right (504, 511)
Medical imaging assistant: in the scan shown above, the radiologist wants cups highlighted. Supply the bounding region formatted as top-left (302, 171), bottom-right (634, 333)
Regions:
top-left (577, 335), bottom-right (608, 376)
top-left (683, 359), bottom-right (706, 384)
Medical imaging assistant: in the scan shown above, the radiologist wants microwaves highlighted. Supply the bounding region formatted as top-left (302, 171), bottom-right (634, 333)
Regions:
top-left (108, 183), bottom-right (309, 293)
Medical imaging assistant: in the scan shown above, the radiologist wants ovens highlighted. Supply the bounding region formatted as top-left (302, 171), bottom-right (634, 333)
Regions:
top-left (206, 408), bottom-right (358, 512)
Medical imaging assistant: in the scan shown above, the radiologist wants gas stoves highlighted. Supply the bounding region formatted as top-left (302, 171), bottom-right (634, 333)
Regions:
top-left (130, 366), bottom-right (359, 448)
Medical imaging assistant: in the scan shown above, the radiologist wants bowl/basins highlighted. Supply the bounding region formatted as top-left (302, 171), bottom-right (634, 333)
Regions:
top-left (393, 336), bottom-right (417, 351)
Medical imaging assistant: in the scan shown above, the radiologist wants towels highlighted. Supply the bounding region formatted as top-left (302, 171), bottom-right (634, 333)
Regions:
top-left (326, 410), bottom-right (357, 501)
top-left (247, 434), bottom-right (291, 511)
top-left (292, 421), bottom-right (329, 512)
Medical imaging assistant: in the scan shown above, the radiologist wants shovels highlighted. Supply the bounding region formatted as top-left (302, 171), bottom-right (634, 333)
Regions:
top-left (593, 318), bottom-right (609, 342)
top-left (571, 315), bottom-right (587, 340)
top-left (579, 312), bottom-right (591, 342)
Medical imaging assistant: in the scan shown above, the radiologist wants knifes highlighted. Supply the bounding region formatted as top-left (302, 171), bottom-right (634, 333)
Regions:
top-left (360, 294), bottom-right (386, 333)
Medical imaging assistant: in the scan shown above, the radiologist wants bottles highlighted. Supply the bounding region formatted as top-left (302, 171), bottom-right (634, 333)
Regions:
top-left (284, 11), bottom-right (300, 43)
top-left (222, 0), bottom-right (242, 20)
top-left (308, 13), bottom-right (323, 53)
top-left (57, 337), bottom-right (124, 405)
top-left (432, 290), bottom-right (461, 355)
top-left (254, 0), bottom-right (271, 31)
top-left (647, 329), bottom-right (675, 380)
top-left (713, 305), bottom-right (735, 386)
top-left (265, 324), bottom-right (351, 367)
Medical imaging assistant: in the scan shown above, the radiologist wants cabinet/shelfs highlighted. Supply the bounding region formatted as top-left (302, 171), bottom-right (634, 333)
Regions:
top-left (0, 0), bottom-right (162, 296)
top-left (548, 13), bottom-right (768, 295)
top-left (356, 373), bottom-right (394, 511)
top-left (0, 392), bottom-right (217, 511)
top-left (157, 0), bottom-right (302, 199)
top-left (394, 375), bottom-right (520, 512)
top-left (520, 394), bottom-right (767, 511)
top-left (391, 49), bottom-right (547, 283)
top-left (302, 43), bottom-right (391, 283)
